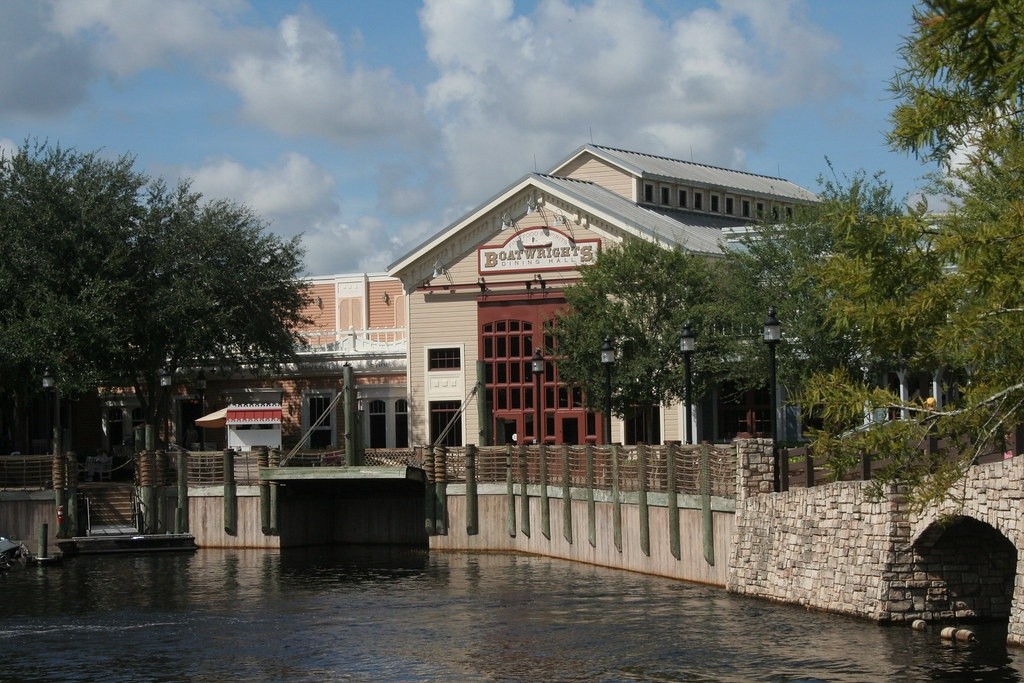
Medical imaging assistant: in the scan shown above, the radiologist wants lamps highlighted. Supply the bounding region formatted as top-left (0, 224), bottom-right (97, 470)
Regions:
top-left (383, 290), bottom-right (389, 304)
top-left (502, 212), bottom-right (515, 230)
top-left (316, 296), bottom-right (324, 307)
top-left (551, 208), bottom-right (573, 227)
top-left (433, 261), bottom-right (457, 285)
top-left (526, 189), bottom-right (542, 216)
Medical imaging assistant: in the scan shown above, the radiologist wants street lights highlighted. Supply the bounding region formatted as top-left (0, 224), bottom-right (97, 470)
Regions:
top-left (762, 308), bottom-right (788, 492)
top-left (601, 338), bottom-right (614, 477)
top-left (679, 323), bottom-right (697, 472)
top-left (532, 349), bottom-right (543, 444)
